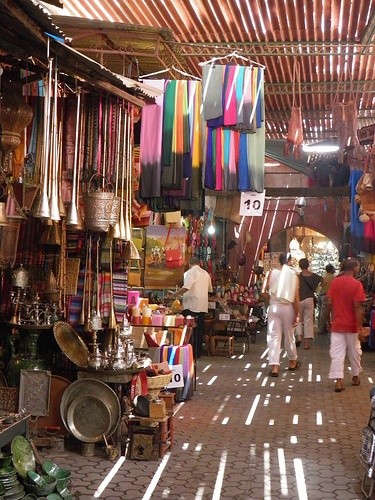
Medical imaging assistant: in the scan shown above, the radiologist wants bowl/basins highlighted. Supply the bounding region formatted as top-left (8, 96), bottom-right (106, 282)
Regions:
top-left (25, 475), bottom-right (56, 497)
top-left (54, 469), bottom-right (71, 487)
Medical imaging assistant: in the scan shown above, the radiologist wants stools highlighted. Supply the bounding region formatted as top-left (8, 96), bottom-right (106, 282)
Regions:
top-left (127, 415), bottom-right (176, 460)
top-left (212, 335), bottom-right (235, 357)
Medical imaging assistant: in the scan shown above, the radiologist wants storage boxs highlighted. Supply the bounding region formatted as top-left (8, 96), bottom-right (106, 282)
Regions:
top-left (126, 291), bottom-right (196, 327)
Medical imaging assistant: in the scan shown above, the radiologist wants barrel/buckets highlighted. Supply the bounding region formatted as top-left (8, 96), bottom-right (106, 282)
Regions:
top-left (81, 172), bottom-right (122, 233)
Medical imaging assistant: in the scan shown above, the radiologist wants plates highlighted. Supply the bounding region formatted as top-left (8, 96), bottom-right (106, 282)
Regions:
top-left (0, 435), bottom-right (74, 500)
top-left (52, 321), bottom-right (120, 442)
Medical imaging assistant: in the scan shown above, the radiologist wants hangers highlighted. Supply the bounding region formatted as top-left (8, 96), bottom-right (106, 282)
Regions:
top-left (138, 62), bottom-right (205, 82)
top-left (198, 49), bottom-right (265, 66)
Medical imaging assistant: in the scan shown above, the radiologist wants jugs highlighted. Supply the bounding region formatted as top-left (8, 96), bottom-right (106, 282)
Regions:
top-left (104, 445), bottom-right (119, 460)
top-left (85, 339), bottom-right (140, 372)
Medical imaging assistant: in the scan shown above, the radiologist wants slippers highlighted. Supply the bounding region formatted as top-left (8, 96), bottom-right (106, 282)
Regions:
top-left (296, 340), bottom-right (301, 347)
top-left (269, 370), bottom-right (278, 377)
top-left (288, 360), bottom-right (302, 371)
top-left (303, 345), bottom-right (310, 349)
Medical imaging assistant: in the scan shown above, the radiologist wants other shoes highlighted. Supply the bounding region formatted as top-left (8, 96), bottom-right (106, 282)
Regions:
top-left (315, 330), bottom-right (323, 334)
top-left (335, 382), bottom-right (345, 393)
top-left (352, 376), bottom-right (360, 386)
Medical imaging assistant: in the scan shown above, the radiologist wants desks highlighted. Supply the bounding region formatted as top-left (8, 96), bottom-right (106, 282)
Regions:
top-left (210, 300), bottom-right (265, 343)
top-left (133, 324), bottom-right (195, 360)
top-left (217, 317), bottom-right (249, 352)
top-left (201, 319), bottom-right (216, 356)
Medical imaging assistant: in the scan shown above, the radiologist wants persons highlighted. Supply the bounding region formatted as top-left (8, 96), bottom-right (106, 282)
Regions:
top-left (316, 263), bottom-right (336, 335)
top-left (326, 259), bottom-right (364, 391)
top-left (172, 257), bottom-right (213, 359)
top-left (295, 258), bottom-right (323, 350)
top-left (261, 252), bottom-right (302, 376)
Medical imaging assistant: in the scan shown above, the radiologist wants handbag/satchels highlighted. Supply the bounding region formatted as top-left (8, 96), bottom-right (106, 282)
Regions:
top-left (253, 248), bottom-right (264, 274)
top-left (356, 153), bottom-right (375, 194)
top-left (314, 295), bottom-right (317, 308)
top-left (284, 106), bottom-right (304, 159)
top-left (166, 248), bottom-right (182, 268)
top-left (332, 101), bottom-right (363, 163)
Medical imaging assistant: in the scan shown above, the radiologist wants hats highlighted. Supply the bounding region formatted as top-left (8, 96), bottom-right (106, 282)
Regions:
top-left (219, 256), bottom-right (228, 270)
top-left (237, 253), bottom-right (246, 266)
